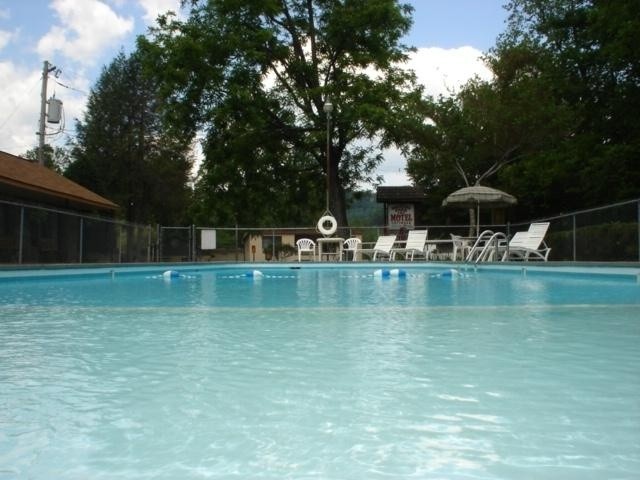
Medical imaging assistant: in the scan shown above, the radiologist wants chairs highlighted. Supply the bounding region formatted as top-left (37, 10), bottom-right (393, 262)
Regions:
top-left (295, 222), bottom-right (552, 263)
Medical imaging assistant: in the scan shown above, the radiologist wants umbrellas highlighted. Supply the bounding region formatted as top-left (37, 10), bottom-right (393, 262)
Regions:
top-left (441, 175), bottom-right (517, 236)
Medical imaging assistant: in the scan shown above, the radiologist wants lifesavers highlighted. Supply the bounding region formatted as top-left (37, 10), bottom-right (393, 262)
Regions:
top-left (317, 216), bottom-right (337, 235)
